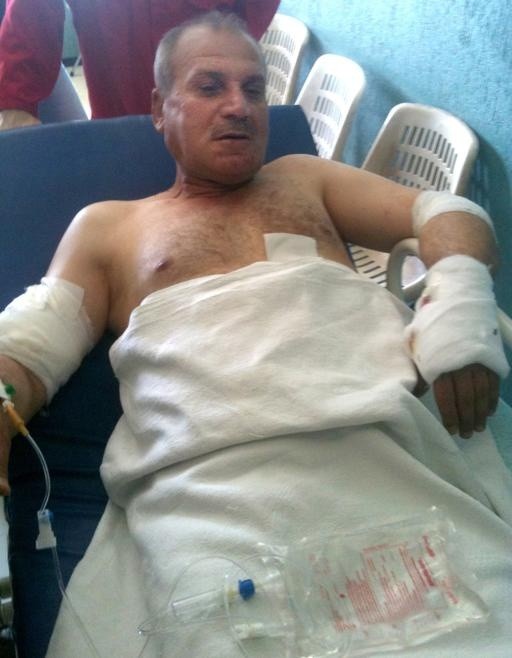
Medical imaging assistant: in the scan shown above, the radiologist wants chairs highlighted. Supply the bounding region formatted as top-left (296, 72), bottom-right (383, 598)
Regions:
top-left (257, 13), bottom-right (310, 106)
top-left (338, 102), bottom-right (479, 303)
top-left (293, 53), bottom-right (366, 159)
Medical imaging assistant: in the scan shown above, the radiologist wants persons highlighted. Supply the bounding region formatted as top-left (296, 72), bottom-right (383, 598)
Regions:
top-left (0, 0), bottom-right (282, 131)
